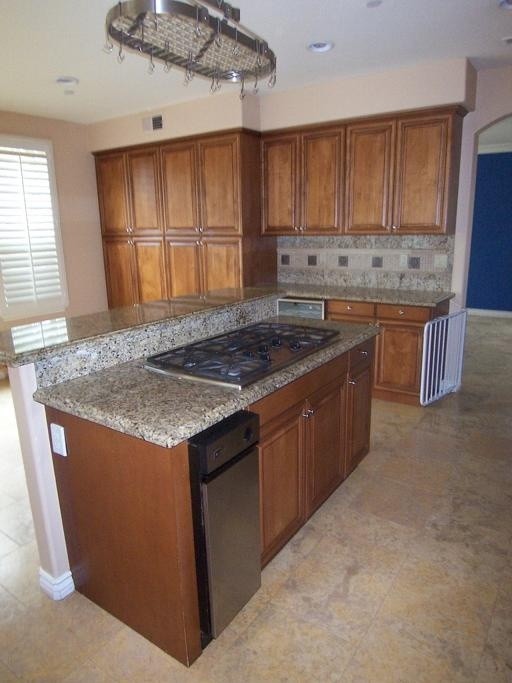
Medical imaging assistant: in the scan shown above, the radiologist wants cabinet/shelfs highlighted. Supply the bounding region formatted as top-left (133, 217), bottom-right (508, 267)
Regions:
top-left (91, 134), bottom-right (276, 310)
top-left (251, 350), bottom-right (347, 570)
top-left (348, 334), bottom-right (377, 476)
top-left (259, 110), bottom-right (463, 236)
top-left (328, 302), bottom-right (450, 407)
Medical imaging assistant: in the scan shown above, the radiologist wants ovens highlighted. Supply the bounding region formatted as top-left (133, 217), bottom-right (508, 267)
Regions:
top-left (189, 410), bottom-right (264, 642)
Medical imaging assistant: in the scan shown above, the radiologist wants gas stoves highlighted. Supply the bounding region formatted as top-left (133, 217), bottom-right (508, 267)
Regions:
top-left (144, 319), bottom-right (344, 392)
